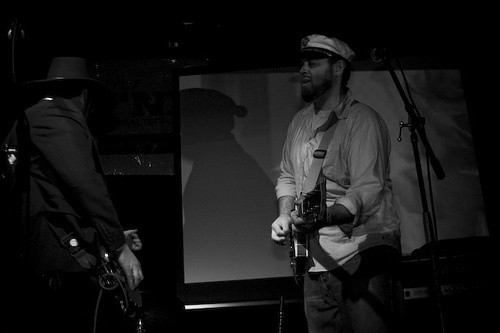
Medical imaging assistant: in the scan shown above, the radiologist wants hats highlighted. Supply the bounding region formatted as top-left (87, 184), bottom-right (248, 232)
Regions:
top-left (23, 56), bottom-right (104, 92)
top-left (300, 34), bottom-right (355, 67)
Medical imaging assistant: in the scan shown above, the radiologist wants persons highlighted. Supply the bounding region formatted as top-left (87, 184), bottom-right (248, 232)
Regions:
top-left (0, 53), bottom-right (145, 333)
top-left (269, 35), bottom-right (403, 332)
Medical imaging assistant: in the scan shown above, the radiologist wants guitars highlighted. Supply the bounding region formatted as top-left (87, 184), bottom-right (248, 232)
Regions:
top-left (285, 178), bottom-right (330, 279)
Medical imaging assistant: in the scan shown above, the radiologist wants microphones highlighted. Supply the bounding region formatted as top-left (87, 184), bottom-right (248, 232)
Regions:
top-left (8, 19), bottom-right (25, 41)
top-left (370, 40), bottom-right (392, 63)
